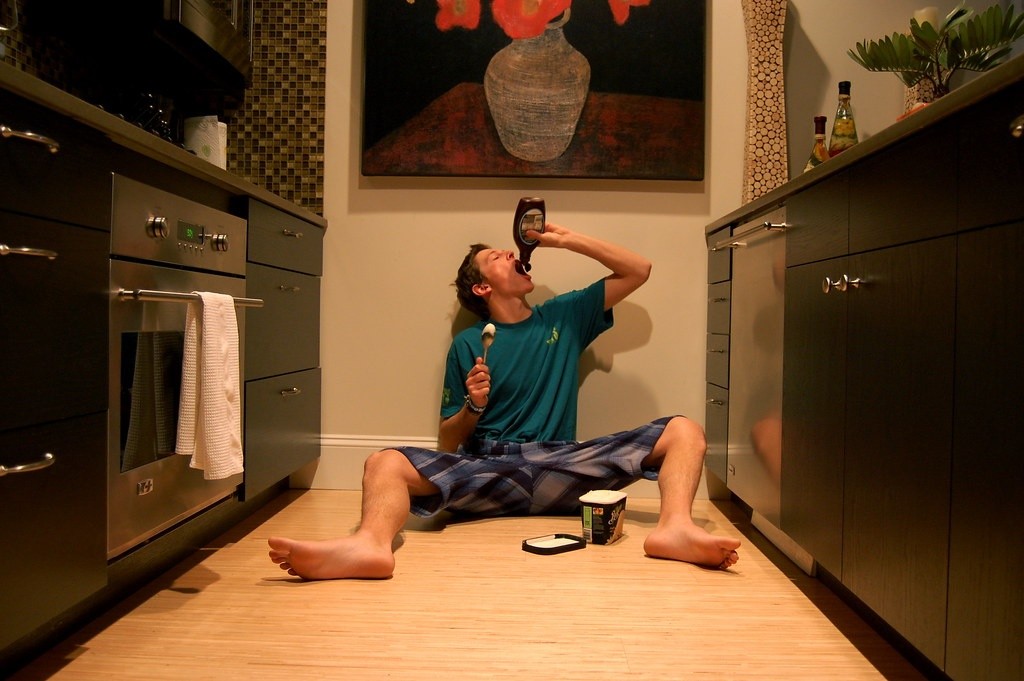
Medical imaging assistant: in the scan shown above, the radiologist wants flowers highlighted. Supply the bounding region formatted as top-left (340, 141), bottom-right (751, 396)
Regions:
top-left (434, 0), bottom-right (652, 39)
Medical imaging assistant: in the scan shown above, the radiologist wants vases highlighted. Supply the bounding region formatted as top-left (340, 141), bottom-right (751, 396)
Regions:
top-left (484, 8), bottom-right (591, 163)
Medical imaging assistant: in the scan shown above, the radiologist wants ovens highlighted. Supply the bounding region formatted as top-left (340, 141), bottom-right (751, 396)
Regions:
top-left (105, 172), bottom-right (264, 566)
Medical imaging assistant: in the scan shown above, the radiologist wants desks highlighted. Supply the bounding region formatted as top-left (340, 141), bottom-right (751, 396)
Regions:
top-left (361, 80), bottom-right (705, 181)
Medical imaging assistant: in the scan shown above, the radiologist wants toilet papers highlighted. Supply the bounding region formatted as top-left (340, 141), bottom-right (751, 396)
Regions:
top-left (184, 113), bottom-right (228, 169)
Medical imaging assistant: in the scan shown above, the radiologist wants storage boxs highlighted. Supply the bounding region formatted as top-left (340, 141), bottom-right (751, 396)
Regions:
top-left (579, 490), bottom-right (629, 544)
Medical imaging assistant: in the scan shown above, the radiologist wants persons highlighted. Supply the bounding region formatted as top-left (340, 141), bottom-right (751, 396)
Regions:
top-left (268, 220), bottom-right (740, 579)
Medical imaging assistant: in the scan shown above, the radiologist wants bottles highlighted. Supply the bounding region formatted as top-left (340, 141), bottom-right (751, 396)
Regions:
top-left (512, 196), bottom-right (546, 274)
top-left (828, 80), bottom-right (859, 158)
top-left (803, 116), bottom-right (831, 173)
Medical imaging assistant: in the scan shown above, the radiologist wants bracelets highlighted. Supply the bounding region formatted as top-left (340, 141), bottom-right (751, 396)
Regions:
top-left (464, 395), bottom-right (486, 416)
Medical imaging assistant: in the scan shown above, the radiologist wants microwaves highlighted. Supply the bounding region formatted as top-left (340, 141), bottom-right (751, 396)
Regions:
top-left (154, 0), bottom-right (254, 96)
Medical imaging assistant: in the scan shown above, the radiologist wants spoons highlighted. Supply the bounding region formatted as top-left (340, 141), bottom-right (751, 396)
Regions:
top-left (482, 323), bottom-right (496, 365)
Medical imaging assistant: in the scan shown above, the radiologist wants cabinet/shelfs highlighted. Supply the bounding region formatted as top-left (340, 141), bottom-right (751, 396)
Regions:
top-left (99, 0), bottom-right (255, 80)
top-left (705, 53), bottom-right (1024, 681)
top-left (0, 60), bottom-right (327, 681)
